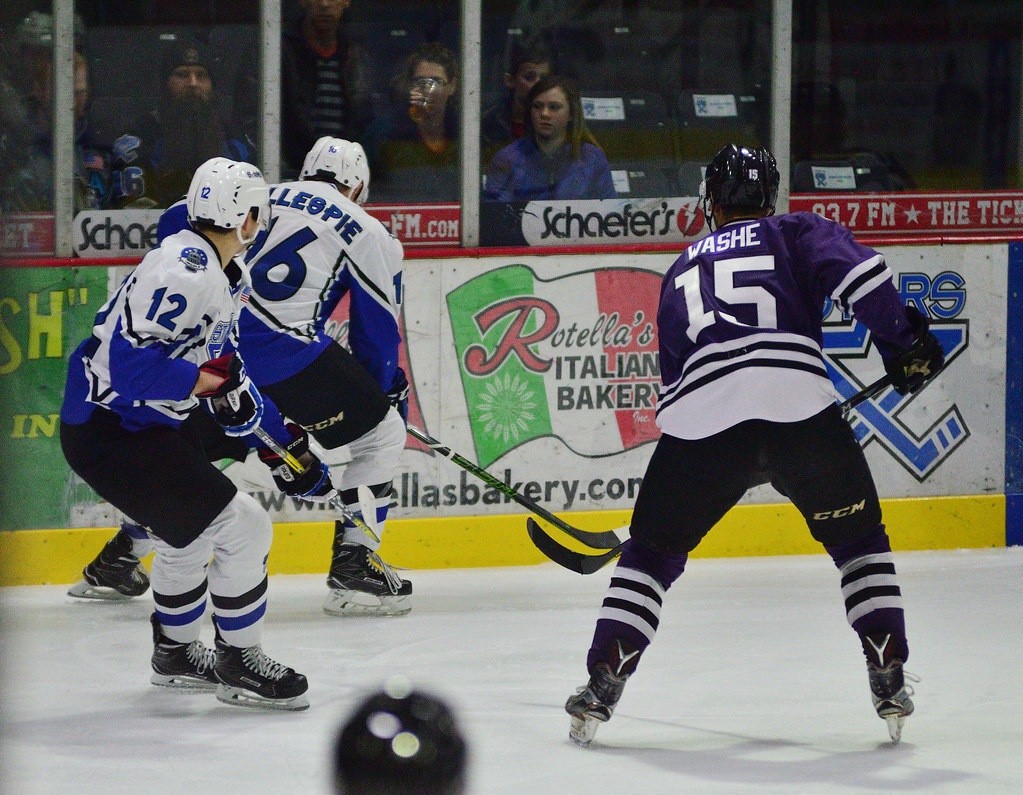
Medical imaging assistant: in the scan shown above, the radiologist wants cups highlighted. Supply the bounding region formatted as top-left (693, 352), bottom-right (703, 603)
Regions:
top-left (408, 78), bottom-right (441, 124)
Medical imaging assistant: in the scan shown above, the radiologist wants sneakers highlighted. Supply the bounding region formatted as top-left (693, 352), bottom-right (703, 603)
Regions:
top-left (326, 518), bottom-right (419, 614)
top-left (866, 657), bottom-right (922, 745)
top-left (65, 528), bottom-right (152, 603)
top-left (207, 614), bottom-right (313, 711)
top-left (564, 658), bottom-right (631, 751)
top-left (147, 611), bottom-right (233, 696)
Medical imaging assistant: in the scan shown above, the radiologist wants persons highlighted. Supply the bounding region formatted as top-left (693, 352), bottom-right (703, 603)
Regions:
top-left (66, 137), bottom-right (413, 615)
top-left (0, 0), bottom-right (463, 212)
top-left (486, 73), bottom-right (617, 201)
top-left (790, 80), bottom-right (922, 196)
top-left (567, 142), bottom-right (945, 747)
top-left (481, 44), bottom-right (555, 140)
top-left (59, 157), bottom-right (312, 710)
top-left (332, 691), bottom-right (466, 795)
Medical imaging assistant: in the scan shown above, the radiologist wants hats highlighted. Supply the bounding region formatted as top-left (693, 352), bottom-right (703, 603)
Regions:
top-left (168, 35), bottom-right (214, 73)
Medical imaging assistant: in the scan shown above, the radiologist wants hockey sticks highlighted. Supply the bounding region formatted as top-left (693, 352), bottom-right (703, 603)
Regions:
top-left (253, 425), bottom-right (382, 545)
top-left (404, 420), bottom-right (632, 551)
top-left (526, 373), bottom-right (891, 576)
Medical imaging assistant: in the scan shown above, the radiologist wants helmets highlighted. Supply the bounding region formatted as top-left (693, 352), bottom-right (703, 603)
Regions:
top-left (295, 135), bottom-right (373, 206)
top-left (186, 155), bottom-right (275, 245)
top-left (695, 142), bottom-right (782, 219)
top-left (331, 684), bottom-right (465, 795)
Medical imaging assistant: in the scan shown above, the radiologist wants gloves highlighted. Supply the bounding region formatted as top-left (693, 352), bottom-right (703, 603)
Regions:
top-left (251, 421), bottom-right (337, 508)
top-left (392, 366), bottom-right (418, 427)
top-left (189, 349), bottom-right (294, 452)
top-left (874, 308), bottom-right (945, 398)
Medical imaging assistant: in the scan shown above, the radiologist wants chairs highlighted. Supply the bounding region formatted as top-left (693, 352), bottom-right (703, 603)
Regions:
top-left (0, 0), bottom-right (1023, 215)
